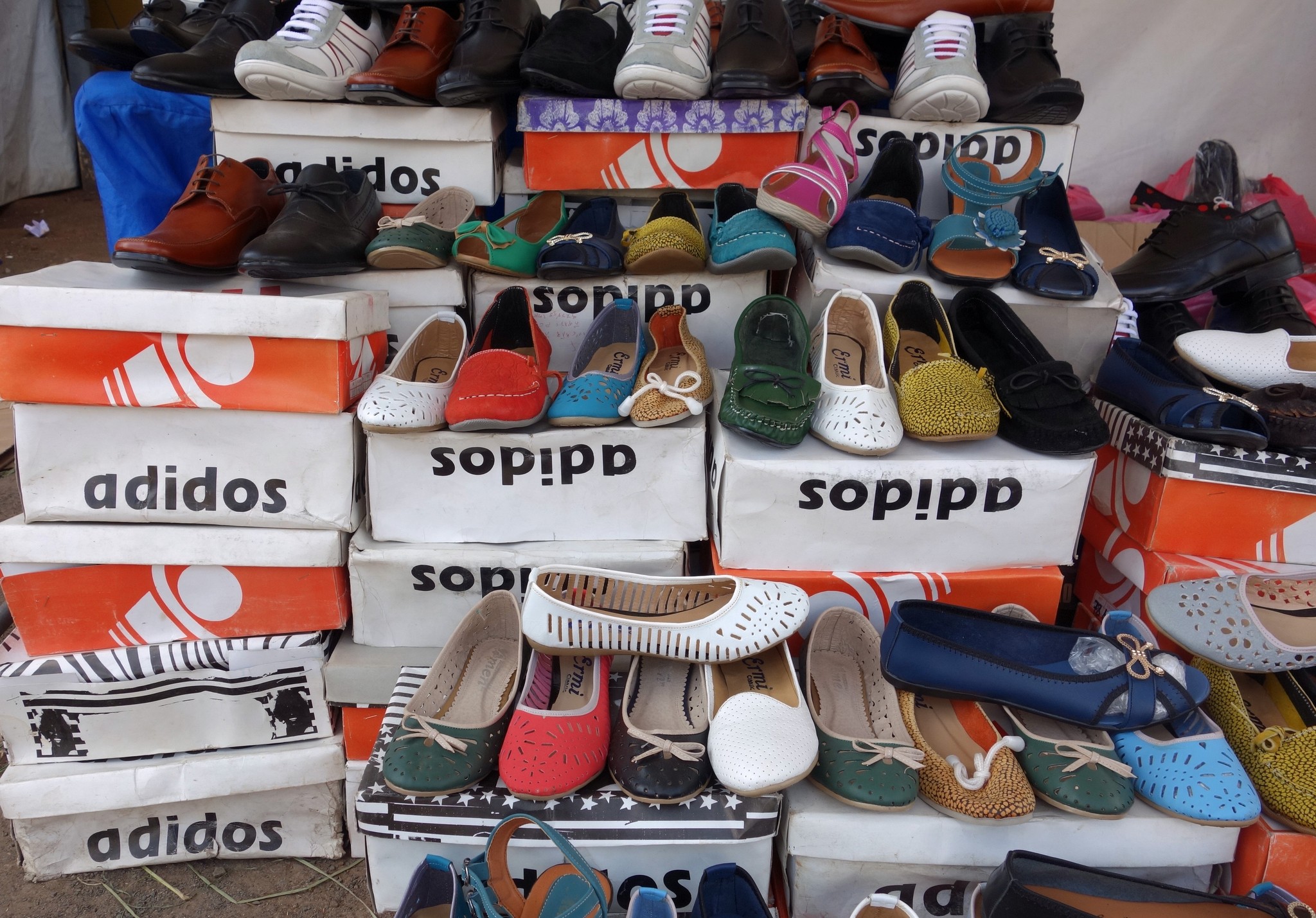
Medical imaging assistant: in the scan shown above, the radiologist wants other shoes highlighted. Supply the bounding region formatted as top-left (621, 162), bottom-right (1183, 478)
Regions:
top-left (710, 181), bottom-right (802, 274)
top-left (356, 277), bottom-right (1120, 457)
top-left (828, 139), bottom-right (933, 271)
top-left (366, 184), bottom-right (477, 267)
top-left (383, 567), bottom-right (1315, 917)
top-left (1006, 172), bottom-right (1099, 298)
top-left (524, 3), bottom-right (637, 96)
top-left (537, 196), bottom-right (627, 273)
top-left (625, 190), bottom-right (711, 273)
top-left (1097, 140), bottom-right (1316, 462)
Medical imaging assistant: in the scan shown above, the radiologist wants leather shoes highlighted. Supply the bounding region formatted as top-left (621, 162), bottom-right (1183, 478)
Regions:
top-left (990, 19), bottom-right (1082, 125)
top-left (131, 0), bottom-right (296, 97)
top-left (68, 0), bottom-right (190, 71)
top-left (803, 0), bottom-right (1055, 34)
top-left (345, 0), bottom-right (467, 106)
top-left (238, 162), bottom-right (384, 275)
top-left (111, 155), bottom-right (291, 276)
top-left (435, 0), bottom-right (543, 103)
top-left (807, 14), bottom-right (891, 109)
top-left (712, 0), bottom-right (801, 99)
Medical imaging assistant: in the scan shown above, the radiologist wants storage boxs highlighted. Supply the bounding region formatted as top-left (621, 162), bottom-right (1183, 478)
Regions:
top-left (0, 100), bottom-right (1316, 918)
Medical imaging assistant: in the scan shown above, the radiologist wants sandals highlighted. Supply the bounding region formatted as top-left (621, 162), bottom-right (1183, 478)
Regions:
top-left (928, 125), bottom-right (1065, 285)
top-left (755, 101), bottom-right (862, 235)
top-left (453, 189), bottom-right (567, 276)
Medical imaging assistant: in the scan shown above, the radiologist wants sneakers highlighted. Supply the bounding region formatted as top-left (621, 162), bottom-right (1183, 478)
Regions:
top-left (614, 0), bottom-right (713, 100)
top-left (891, 11), bottom-right (989, 122)
top-left (233, 0), bottom-right (386, 101)
